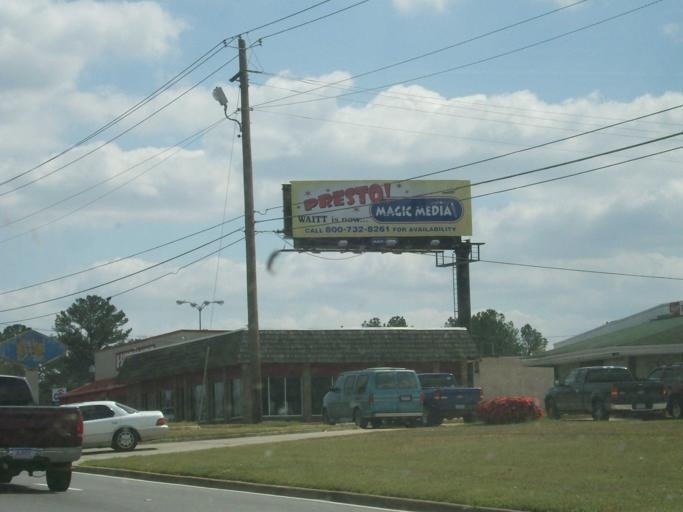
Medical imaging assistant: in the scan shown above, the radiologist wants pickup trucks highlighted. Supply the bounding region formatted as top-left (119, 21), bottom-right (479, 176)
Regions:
top-left (1, 375), bottom-right (85, 491)
top-left (544, 365), bottom-right (664, 422)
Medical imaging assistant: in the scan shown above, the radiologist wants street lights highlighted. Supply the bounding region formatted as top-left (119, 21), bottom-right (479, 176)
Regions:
top-left (176, 299), bottom-right (227, 330)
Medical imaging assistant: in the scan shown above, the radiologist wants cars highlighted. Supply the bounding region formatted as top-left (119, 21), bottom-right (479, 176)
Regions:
top-left (52, 400), bottom-right (168, 450)
top-left (646, 363), bottom-right (683, 421)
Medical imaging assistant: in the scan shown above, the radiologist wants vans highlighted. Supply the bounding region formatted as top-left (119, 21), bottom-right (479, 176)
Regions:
top-left (318, 366), bottom-right (425, 428)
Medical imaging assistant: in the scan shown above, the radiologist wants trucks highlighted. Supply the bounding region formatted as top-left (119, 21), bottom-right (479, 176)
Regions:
top-left (415, 373), bottom-right (483, 426)
